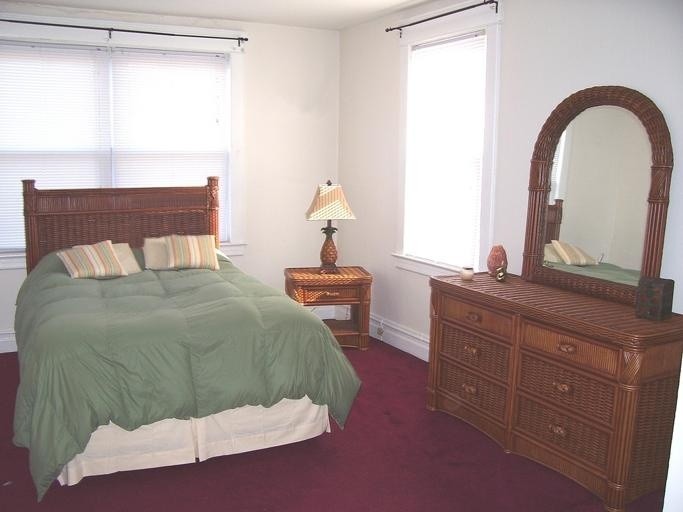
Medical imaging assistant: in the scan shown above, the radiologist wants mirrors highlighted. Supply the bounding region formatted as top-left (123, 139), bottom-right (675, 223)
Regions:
top-left (521, 86), bottom-right (673, 309)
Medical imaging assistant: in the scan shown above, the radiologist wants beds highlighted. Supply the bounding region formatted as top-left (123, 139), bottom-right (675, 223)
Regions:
top-left (11, 177), bottom-right (363, 504)
top-left (543, 198), bottom-right (640, 287)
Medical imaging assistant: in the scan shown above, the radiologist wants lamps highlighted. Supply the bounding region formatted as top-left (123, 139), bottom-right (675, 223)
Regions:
top-left (305, 179), bottom-right (356, 274)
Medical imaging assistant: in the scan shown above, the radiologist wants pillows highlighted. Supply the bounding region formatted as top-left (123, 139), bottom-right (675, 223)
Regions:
top-left (166, 234), bottom-right (221, 271)
top-left (543, 243), bottom-right (563, 265)
top-left (142, 238), bottom-right (173, 271)
top-left (111, 243), bottom-right (142, 275)
top-left (56, 239), bottom-right (128, 279)
top-left (551, 239), bottom-right (597, 267)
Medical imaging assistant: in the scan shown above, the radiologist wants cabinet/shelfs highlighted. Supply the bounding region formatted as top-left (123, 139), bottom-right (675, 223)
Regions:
top-left (425, 271), bottom-right (683, 512)
top-left (284, 266), bottom-right (373, 352)
